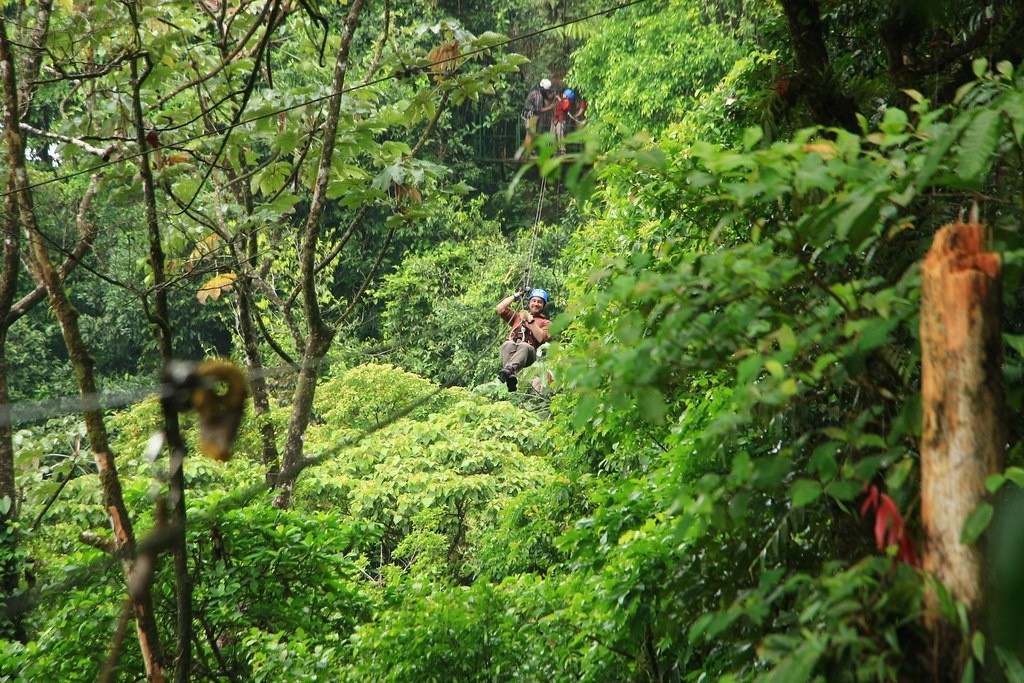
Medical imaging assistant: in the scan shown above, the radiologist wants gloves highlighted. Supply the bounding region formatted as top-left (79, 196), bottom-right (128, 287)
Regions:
top-left (519, 309), bottom-right (533, 323)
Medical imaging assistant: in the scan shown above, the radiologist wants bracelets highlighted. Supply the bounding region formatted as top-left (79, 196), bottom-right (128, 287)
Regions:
top-left (528, 320), bottom-right (535, 323)
top-left (513, 294), bottom-right (520, 301)
top-left (577, 114), bottom-right (579, 117)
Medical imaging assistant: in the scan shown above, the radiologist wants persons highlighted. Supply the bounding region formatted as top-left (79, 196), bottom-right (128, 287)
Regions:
top-left (575, 100), bottom-right (586, 118)
top-left (516, 79), bottom-right (555, 160)
top-left (550, 89), bottom-right (574, 155)
top-left (496, 289), bottom-right (552, 392)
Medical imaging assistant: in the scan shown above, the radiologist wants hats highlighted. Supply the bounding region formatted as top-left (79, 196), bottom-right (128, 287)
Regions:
top-left (539, 79), bottom-right (552, 89)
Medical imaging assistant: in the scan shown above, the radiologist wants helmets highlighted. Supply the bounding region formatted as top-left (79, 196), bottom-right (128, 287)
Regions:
top-left (564, 89), bottom-right (574, 100)
top-left (529, 289), bottom-right (548, 304)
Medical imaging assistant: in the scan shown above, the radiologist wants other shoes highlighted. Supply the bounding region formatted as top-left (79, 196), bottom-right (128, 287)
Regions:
top-left (507, 374), bottom-right (517, 392)
top-left (498, 366), bottom-right (514, 383)
